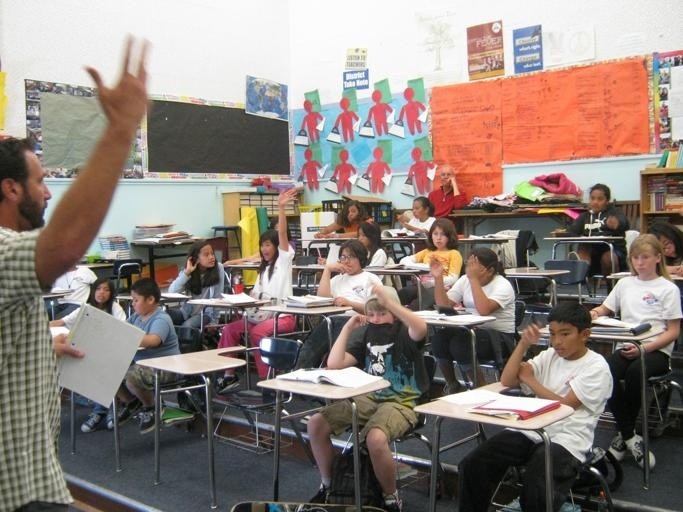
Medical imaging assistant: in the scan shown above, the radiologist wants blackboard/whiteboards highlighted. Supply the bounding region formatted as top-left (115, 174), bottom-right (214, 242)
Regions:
top-left (141, 94), bottom-right (293, 183)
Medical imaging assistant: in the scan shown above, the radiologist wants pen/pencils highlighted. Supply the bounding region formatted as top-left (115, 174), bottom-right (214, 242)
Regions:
top-left (317, 248), bottom-right (323, 265)
top-left (549, 229), bottom-right (566, 234)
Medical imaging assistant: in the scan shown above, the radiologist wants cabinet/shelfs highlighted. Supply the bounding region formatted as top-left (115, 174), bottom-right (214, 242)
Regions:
top-left (639, 167), bottom-right (682, 285)
top-left (323, 197), bottom-right (391, 229)
top-left (221, 191), bottom-right (308, 263)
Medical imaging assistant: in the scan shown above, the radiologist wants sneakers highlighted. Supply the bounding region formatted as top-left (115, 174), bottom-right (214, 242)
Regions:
top-left (309, 482), bottom-right (330, 504)
top-left (608, 432), bottom-right (627, 461)
top-left (444, 382), bottom-right (462, 395)
top-left (384, 494), bottom-right (402, 512)
top-left (106, 396), bottom-right (167, 434)
top-left (625, 429), bottom-right (656, 470)
top-left (217, 376), bottom-right (240, 394)
top-left (81, 414), bottom-right (104, 432)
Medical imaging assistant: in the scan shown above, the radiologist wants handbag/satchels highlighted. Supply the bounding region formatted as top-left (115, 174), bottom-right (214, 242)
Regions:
top-left (246, 291), bottom-right (277, 324)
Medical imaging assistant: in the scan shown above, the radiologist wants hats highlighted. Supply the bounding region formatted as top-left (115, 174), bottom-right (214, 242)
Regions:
top-left (363, 286), bottom-right (401, 316)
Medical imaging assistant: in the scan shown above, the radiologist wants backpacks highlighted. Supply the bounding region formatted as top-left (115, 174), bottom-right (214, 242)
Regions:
top-left (327, 445), bottom-right (384, 508)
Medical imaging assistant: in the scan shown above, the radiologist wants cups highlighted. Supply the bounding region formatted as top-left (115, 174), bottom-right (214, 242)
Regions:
top-left (256, 186), bottom-right (266, 193)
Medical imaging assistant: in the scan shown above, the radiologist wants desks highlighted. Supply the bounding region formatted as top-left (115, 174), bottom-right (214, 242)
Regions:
top-left (42, 289), bottom-right (76, 320)
top-left (74, 260), bottom-right (114, 272)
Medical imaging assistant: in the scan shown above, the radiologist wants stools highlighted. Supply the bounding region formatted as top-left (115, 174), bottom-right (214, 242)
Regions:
top-left (211, 224), bottom-right (242, 264)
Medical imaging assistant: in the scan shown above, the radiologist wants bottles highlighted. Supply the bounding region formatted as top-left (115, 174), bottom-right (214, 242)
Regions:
top-left (231, 274), bottom-right (245, 295)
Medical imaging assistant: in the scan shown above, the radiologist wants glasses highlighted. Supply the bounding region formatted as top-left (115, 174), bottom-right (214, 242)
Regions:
top-left (663, 240), bottom-right (674, 252)
top-left (339, 255), bottom-right (357, 261)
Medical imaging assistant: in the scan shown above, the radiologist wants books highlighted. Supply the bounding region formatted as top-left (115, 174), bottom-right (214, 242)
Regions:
top-left (284, 294), bottom-right (336, 309)
top-left (648, 143), bottom-right (683, 229)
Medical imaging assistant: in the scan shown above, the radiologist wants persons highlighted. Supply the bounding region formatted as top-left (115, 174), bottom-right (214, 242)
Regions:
top-left (51, 183), bottom-right (682, 511)
top-left (0, 34), bottom-right (156, 512)
top-left (394, 166), bottom-right (466, 257)
